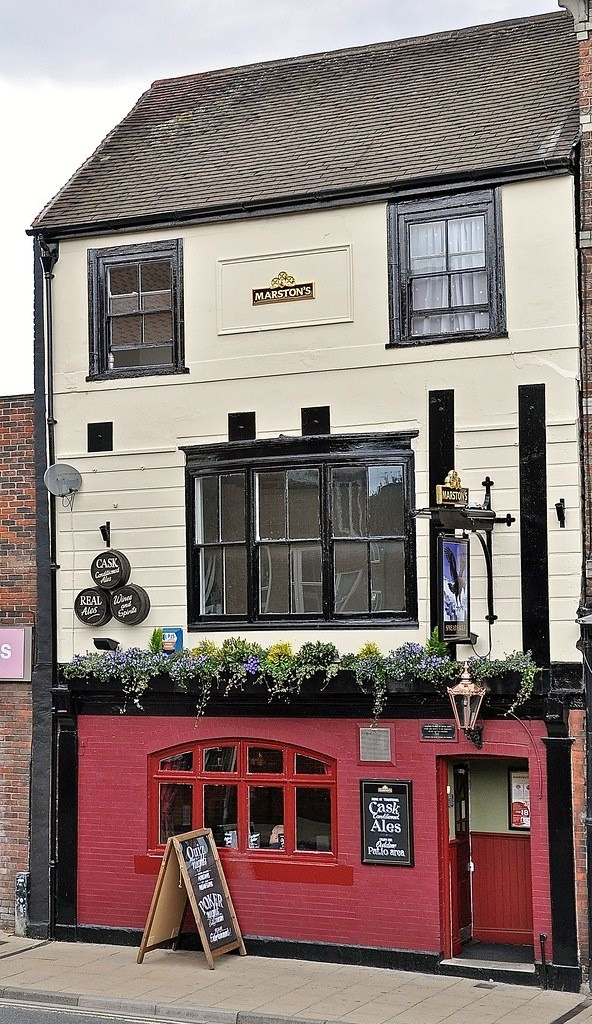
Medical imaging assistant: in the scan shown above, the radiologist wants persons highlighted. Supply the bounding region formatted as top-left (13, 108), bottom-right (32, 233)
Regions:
top-left (268, 824), bottom-right (285, 848)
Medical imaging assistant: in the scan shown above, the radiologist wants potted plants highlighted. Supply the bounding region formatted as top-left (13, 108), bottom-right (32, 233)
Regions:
top-left (61, 626), bottom-right (548, 726)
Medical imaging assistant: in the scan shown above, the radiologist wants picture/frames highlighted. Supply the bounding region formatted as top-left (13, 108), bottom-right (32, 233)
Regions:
top-left (359, 778), bottom-right (414, 867)
top-left (507, 767), bottom-right (531, 831)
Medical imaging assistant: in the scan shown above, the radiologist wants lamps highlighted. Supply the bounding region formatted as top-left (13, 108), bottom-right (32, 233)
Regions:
top-left (447, 661), bottom-right (488, 751)
